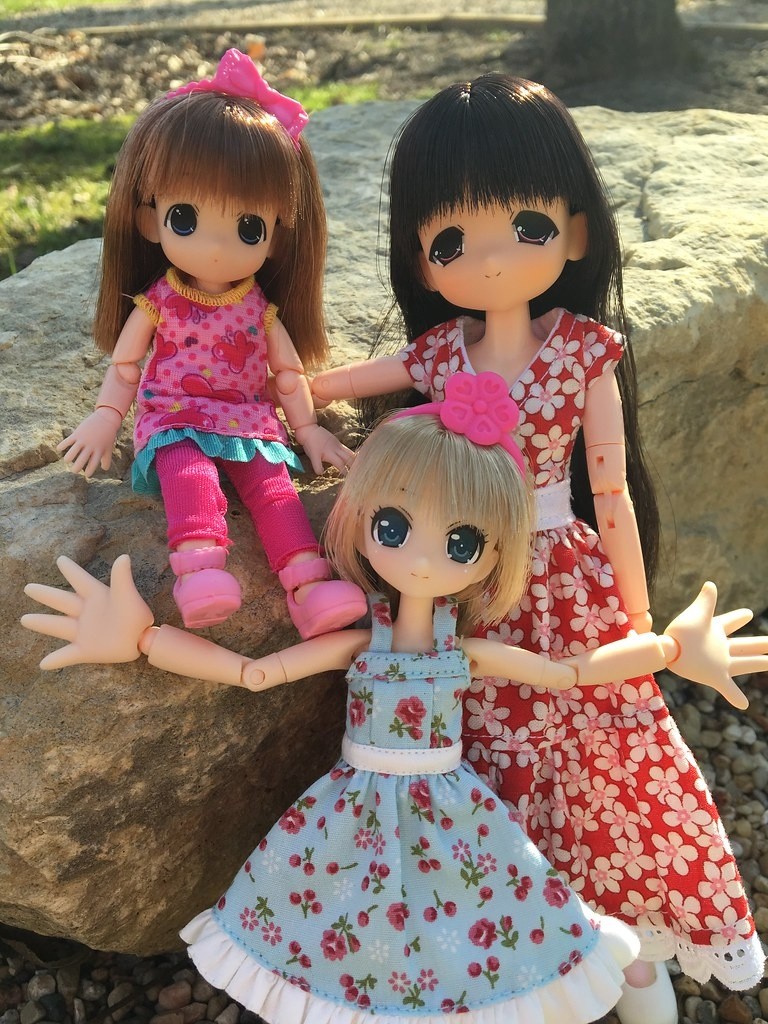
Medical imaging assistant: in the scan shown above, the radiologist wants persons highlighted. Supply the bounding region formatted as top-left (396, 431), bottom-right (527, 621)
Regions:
top-left (54, 47), bottom-right (366, 640)
top-left (21, 371), bottom-right (768, 1024)
top-left (270, 78), bottom-right (766, 1024)
